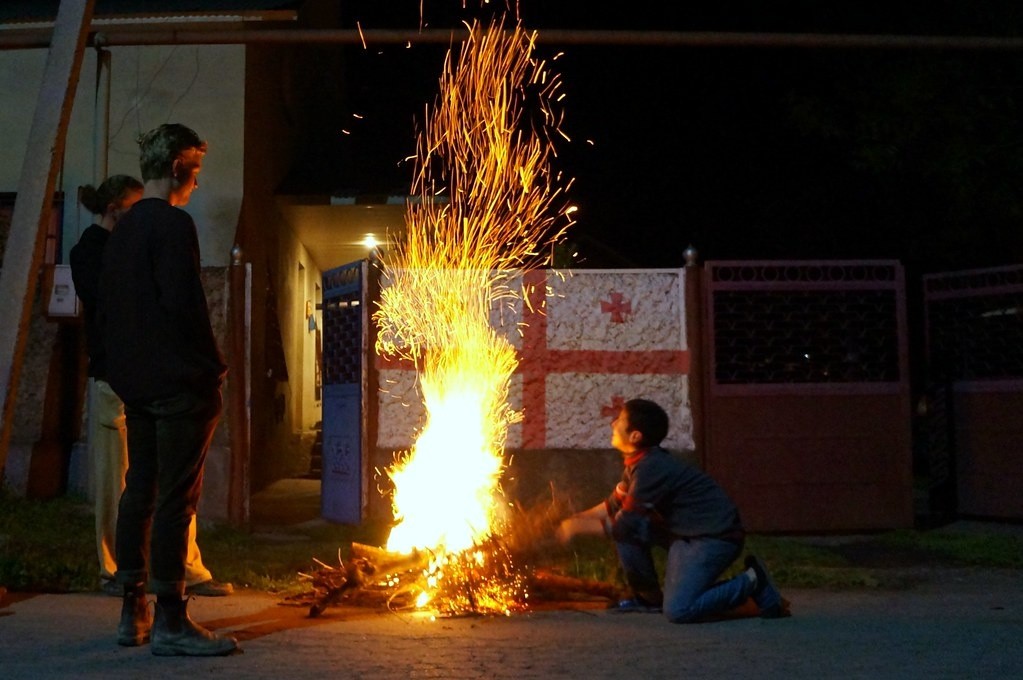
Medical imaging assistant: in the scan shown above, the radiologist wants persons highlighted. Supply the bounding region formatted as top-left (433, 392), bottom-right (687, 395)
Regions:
top-left (554, 399), bottom-right (787, 624)
top-left (96, 123), bottom-right (237, 656)
top-left (65, 175), bottom-right (234, 596)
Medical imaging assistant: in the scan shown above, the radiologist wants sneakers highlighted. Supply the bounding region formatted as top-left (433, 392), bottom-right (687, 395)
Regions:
top-left (608, 591), bottom-right (663, 613)
top-left (743, 553), bottom-right (788, 617)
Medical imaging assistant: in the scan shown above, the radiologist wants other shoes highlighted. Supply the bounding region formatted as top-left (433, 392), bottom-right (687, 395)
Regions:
top-left (185, 579), bottom-right (233, 596)
top-left (100, 577), bottom-right (124, 596)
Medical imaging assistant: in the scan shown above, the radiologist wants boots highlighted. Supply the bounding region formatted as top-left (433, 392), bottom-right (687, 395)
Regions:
top-left (117, 591), bottom-right (154, 646)
top-left (146, 596), bottom-right (238, 656)
